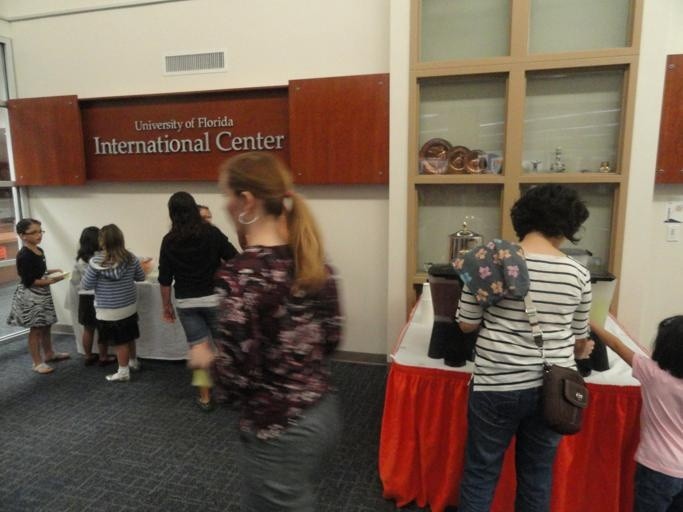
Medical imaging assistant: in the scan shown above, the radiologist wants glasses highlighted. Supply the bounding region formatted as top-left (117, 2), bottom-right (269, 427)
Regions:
top-left (24, 231), bottom-right (45, 235)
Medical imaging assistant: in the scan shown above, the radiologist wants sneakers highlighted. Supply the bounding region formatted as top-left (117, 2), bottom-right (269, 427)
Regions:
top-left (98, 356), bottom-right (116, 366)
top-left (129, 360), bottom-right (143, 372)
top-left (192, 396), bottom-right (212, 411)
top-left (44, 351), bottom-right (69, 363)
top-left (32, 362), bottom-right (54, 374)
top-left (105, 371), bottom-right (130, 382)
top-left (84, 352), bottom-right (100, 366)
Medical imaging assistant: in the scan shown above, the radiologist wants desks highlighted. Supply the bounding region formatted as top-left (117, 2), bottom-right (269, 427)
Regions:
top-left (374, 283), bottom-right (653, 512)
top-left (64, 274), bottom-right (219, 363)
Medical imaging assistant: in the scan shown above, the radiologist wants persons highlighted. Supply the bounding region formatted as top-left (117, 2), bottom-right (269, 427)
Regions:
top-left (80, 223), bottom-right (146, 382)
top-left (215, 152), bottom-right (342, 511)
top-left (72, 226), bottom-right (117, 366)
top-left (457, 184), bottom-right (592, 512)
top-left (157, 191), bottom-right (241, 411)
top-left (196, 206), bottom-right (211, 225)
top-left (589, 316), bottom-right (683, 511)
top-left (6, 219), bottom-right (70, 374)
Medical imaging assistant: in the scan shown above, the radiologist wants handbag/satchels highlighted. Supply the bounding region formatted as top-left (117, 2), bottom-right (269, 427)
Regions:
top-left (520, 294), bottom-right (589, 435)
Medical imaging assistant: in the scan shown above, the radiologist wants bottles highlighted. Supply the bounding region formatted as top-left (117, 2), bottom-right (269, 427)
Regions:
top-left (549, 146), bottom-right (566, 173)
top-left (599, 160), bottom-right (611, 173)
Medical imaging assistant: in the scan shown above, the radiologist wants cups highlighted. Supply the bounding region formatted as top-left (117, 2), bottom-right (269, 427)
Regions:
top-left (590, 256), bottom-right (602, 274)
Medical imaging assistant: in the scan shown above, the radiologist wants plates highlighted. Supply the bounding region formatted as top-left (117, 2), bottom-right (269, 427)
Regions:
top-left (444, 145), bottom-right (472, 175)
top-left (418, 137), bottom-right (450, 175)
top-left (483, 153), bottom-right (503, 174)
top-left (463, 150), bottom-right (487, 173)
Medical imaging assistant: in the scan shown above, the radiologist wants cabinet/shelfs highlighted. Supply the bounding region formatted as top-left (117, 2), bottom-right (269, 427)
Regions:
top-left (406, 1), bottom-right (645, 320)
